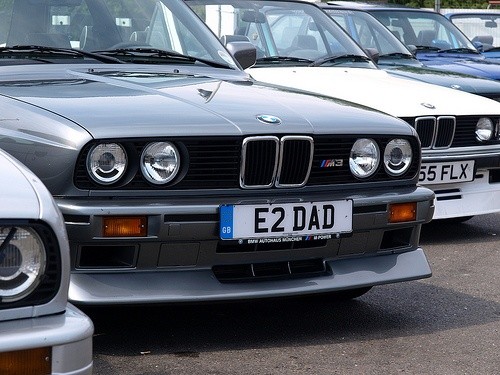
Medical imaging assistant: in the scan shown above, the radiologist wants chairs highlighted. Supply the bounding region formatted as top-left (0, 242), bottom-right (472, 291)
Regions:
top-left (417, 30), bottom-right (437, 46)
top-left (288, 35), bottom-right (318, 53)
top-left (80, 25), bottom-right (127, 51)
top-left (220, 35), bottom-right (251, 48)
top-left (23, 32), bottom-right (71, 50)
top-left (129, 31), bottom-right (164, 48)
top-left (470, 35), bottom-right (493, 48)
top-left (377, 30), bottom-right (401, 49)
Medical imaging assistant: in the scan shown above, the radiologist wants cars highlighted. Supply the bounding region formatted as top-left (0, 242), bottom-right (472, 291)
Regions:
top-left (147, 0), bottom-right (500, 221)
top-left (312, 0), bottom-right (500, 79)
top-left (432, 6), bottom-right (500, 49)
top-left (0, 0), bottom-right (437, 306)
top-left (245, 7), bottom-right (500, 104)
top-left (1, 147), bottom-right (93, 375)
